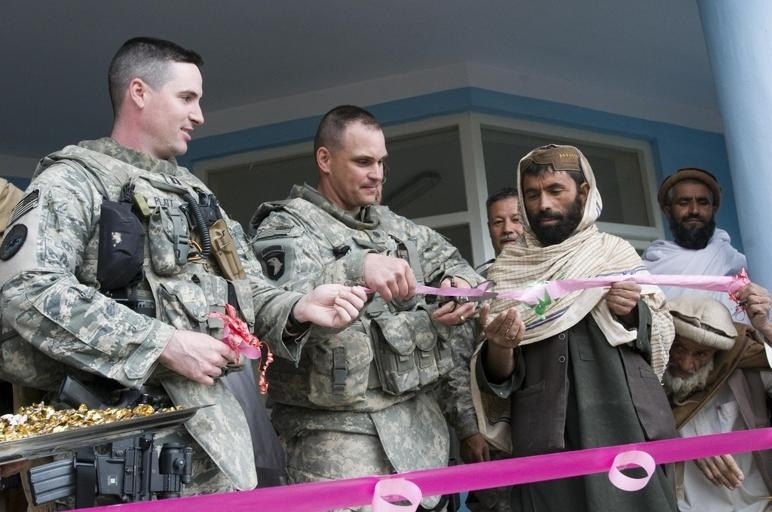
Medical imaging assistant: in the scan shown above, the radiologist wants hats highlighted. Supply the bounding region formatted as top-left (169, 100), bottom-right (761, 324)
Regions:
top-left (663, 294), bottom-right (738, 351)
top-left (519, 145), bottom-right (582, 174)
top-left (659, 169), bottom-right (722, 210)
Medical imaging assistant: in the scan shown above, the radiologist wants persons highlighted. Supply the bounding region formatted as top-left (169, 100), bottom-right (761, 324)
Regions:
top-left (635, 167), bottom-right (772, 512)
top-left (477, 147), bottom-right (674, 511)
top-left (449, 187), bottom-right (527, 510)
top-left (250, 106), bottom-right (469, 512)
top-left (653, 282), bottom-right (771, 492)
top-left (1, 36), bottom-right (366, 512)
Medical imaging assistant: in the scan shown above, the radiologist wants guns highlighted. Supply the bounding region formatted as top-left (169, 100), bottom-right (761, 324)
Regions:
top-left (174, 188), bottom-right (250, 327)
top-left (27, 428), bottom-right (194, 507)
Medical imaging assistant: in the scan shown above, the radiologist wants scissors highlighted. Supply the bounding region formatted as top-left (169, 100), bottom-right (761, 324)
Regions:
top-left (437, 276), bottom-right (498, 312)
